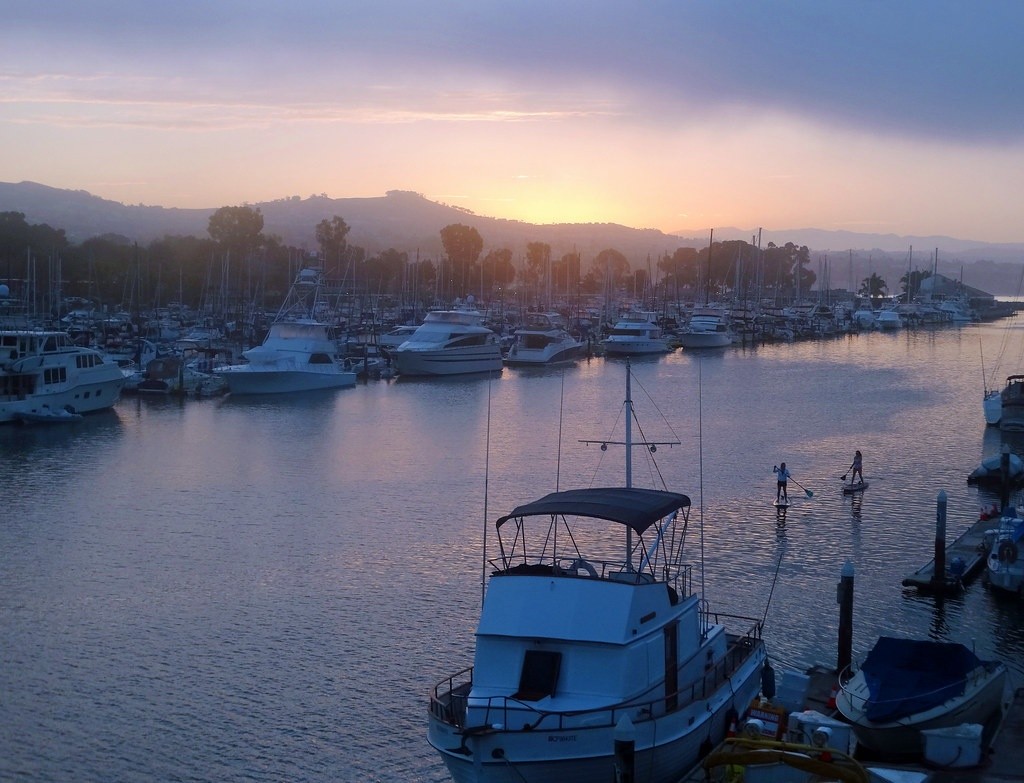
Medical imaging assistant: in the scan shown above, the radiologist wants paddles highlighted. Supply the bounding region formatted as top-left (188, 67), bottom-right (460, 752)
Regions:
top-left (840, 464), bottom-right (854, 481)
top-left (774, 465), bottom-right (814, 498)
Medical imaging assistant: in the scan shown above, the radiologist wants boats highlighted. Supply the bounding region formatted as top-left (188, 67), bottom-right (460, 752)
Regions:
top-left (420, 350), bottom-right (777, 783)
top-left (0, 228), bottom-right (1024, 783)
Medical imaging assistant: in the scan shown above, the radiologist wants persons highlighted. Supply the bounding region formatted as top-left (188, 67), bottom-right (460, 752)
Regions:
top-left (773, 462), bottom-right (791, 503)
top-left (850, 451), bottom-right (864, 486)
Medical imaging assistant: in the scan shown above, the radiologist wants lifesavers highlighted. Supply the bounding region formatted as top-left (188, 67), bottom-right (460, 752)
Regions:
top-left (998, 541), bottom-right (1018, 563)
